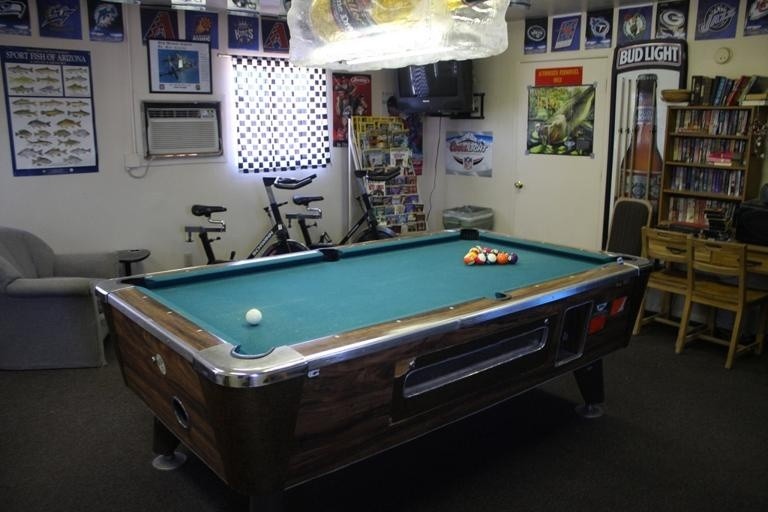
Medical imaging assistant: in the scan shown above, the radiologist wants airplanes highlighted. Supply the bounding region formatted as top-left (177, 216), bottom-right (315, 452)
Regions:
top-left (157, 53), bottom-right (191, 82)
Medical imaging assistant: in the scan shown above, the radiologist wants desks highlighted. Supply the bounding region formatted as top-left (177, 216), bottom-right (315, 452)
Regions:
top-left (641, 237), bottom-right (768, 312)
top-left (95, 227), bottom-right (653, 512)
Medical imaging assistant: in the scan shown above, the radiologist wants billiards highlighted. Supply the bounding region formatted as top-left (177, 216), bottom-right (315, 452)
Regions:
top-left (464, 246), bottom-right (518, 265)
top-left (246, 309), bottom-right (261, 325)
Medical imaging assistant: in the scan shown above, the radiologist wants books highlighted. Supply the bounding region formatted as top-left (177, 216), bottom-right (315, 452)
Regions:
top-left (345, 116), bottom-right (427, 233)
top-left (661, 74), bottom-right (768, 240)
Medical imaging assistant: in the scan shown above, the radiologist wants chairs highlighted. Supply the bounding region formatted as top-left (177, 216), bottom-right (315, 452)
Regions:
top-left (0, 228), bottom-right (144, 370)
top-left (676, 236), bottom-right (768, 369)
top-left (632, 227), bottom-right (710, 345)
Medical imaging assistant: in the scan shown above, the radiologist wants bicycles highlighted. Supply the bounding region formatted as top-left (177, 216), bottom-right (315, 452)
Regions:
top-left (183, 174), bottom-right (318, 265)
top-left (284, 166), bottom-right (404, 247)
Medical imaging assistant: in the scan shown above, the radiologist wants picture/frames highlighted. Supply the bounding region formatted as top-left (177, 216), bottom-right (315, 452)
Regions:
top-left (147, 37), bottom-right (213, 94)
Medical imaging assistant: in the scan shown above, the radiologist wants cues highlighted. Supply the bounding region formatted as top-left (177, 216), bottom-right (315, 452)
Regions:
top-left (618, 78), bottom-right (657, 201)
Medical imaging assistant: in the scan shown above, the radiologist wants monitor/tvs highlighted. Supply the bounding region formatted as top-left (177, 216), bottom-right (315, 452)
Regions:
top-left (394, 61), bottom-right (472, 115)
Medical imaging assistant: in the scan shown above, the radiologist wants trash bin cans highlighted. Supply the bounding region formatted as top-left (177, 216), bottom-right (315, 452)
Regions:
top-left (443, 206), bottom-right (494, 232)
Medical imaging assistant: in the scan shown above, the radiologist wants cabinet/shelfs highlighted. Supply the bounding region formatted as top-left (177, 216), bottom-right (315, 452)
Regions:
top-left (658, 106), bottom-right (768, 232)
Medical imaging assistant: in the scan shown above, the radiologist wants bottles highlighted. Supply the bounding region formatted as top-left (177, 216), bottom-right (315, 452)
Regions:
top-left (539, 85), bottom-right (595, 146)
top-left (615, 74), bottom-right (663, 227)
